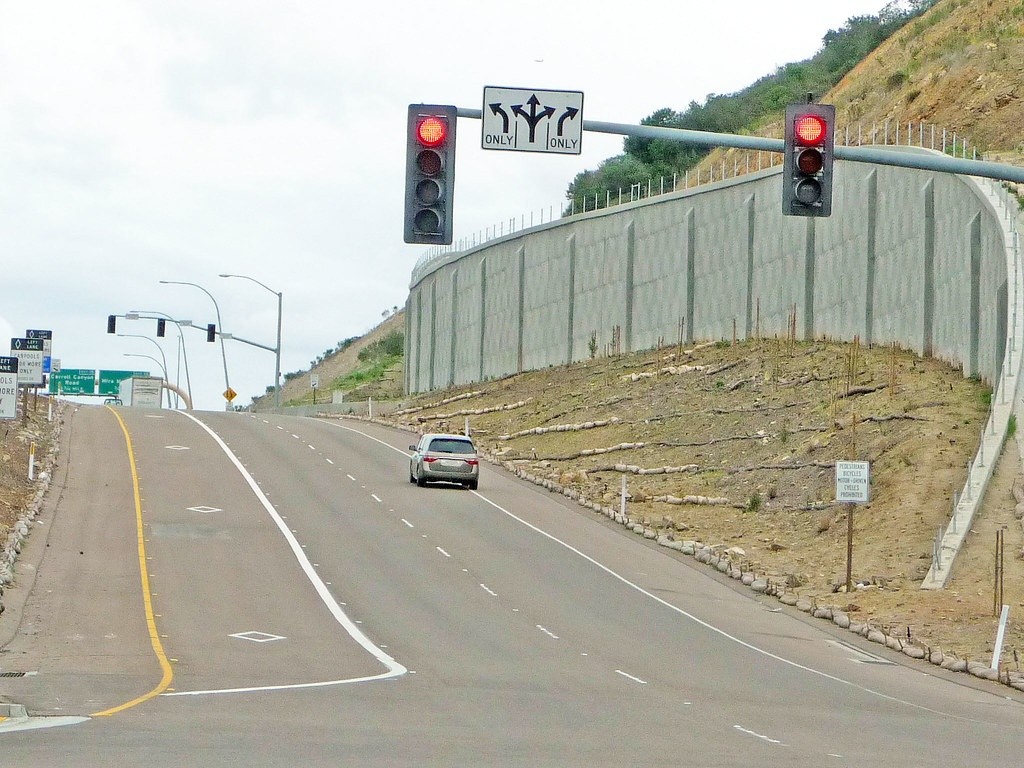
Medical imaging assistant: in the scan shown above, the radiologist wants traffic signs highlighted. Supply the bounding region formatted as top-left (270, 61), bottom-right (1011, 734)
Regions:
top-left (480, 84), bottom-right (585, 155)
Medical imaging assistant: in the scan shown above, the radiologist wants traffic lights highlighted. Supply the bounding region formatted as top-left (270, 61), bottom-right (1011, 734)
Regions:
top-left (157, 318), bottom-right (165, 337)
top-left (207, 324), bottom-right (216, 342)
top-left (404, 104), bottom-right (458, 245)
top-left (107, 315), bottom-right (116, 334)
top-left (783, 103), bottom-right (836, 217)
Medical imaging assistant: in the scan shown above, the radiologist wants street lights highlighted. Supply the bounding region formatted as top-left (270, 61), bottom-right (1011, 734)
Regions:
top-left (219, 274), bottom-right (283, 407)
top-left (159, 280), bottom-right (231, 411)
top-left (129, 311), bottom-right (193, 409)
top-left (117, 334), bottom-right (171, 408)
top-left (124, 353), bottom-right (165, 377)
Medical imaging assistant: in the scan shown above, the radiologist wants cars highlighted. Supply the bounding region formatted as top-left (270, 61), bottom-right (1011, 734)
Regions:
top-left (409, 433), bottom-right (479, 490)
